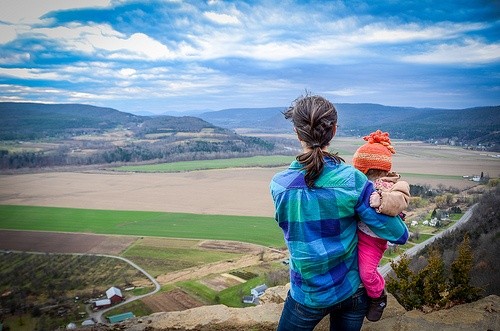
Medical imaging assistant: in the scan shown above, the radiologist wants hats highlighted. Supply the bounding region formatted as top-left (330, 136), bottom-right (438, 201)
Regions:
top-left (352, 130), bottom-right (396, 174)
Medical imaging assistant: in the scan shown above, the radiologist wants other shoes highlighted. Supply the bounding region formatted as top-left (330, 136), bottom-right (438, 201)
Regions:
top-left (366, 288), bottom-right (387, 322)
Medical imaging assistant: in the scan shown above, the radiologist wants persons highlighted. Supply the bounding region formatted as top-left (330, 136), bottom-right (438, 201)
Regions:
top-left (268, 89), bottom-right (409, 331)
top-left (351, 130), bottom-right (412, 322)
